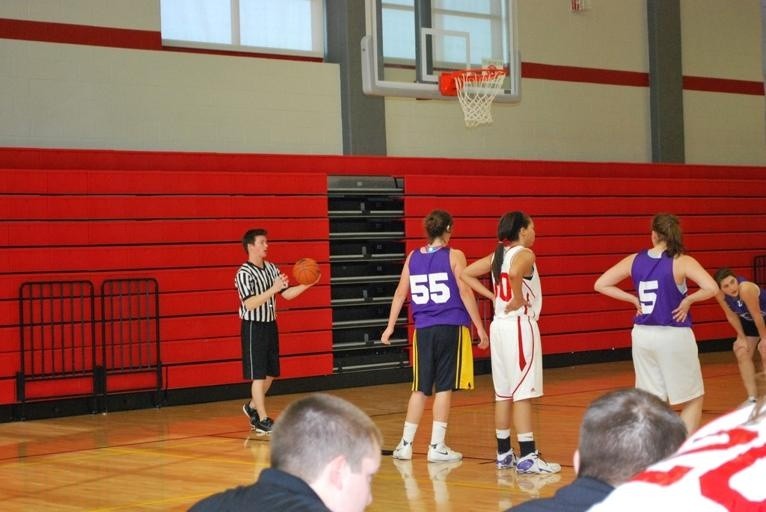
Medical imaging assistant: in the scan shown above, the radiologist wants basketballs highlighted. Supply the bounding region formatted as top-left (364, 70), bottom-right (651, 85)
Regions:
top-left (293, 258), bottom-right (319, 284)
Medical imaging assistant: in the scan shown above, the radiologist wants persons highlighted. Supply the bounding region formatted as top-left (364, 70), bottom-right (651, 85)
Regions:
top-left (186, 392), bottom-right (382, 512)
top-left (594, 212), bottom-right (719, 442)
top-left (496, 468), bottom-right (560, 511)
top-left (381, 210), bottom-right (489, 463)
top-left (234, 229), bottom-right (321, 434)
top-left (714, 268), bottom-right (766, 403)
top-left (504, 388), bottom-right (688, 512)
top-left (392, 458), bottom-right (463, 512)
top-left (459, 210), bottom-right (561, 475)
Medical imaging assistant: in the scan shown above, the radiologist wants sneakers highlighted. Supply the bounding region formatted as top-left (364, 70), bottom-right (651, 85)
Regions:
top-left (392, 439), bottom-right (413, 459)
top-left (427, 442), bottom-right (462, 462)
top-left (243, 400), bottom-right (273, 433)
top-left (496, 448), bottom-right (561, 474)
